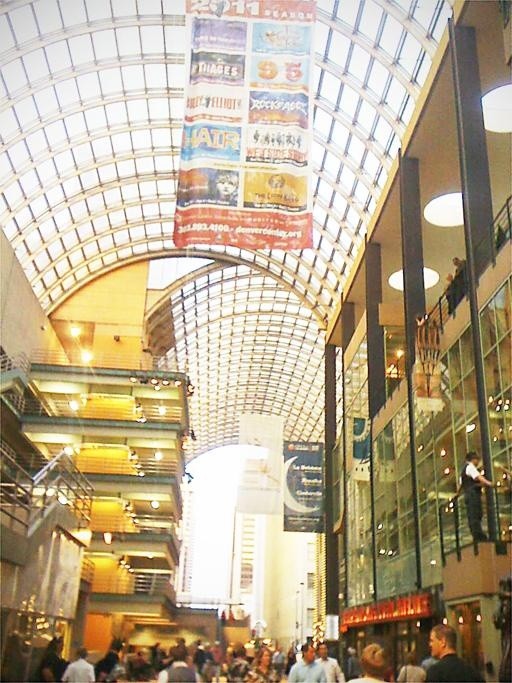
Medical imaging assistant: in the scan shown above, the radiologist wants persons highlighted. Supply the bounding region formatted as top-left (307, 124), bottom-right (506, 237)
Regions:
top-left (221, 611), bottom-right (226, 620)
top-left (33, 626), bottom-right (481, 683)
top-left (443, 274), bottom-right (460, 313)
top-left (228, 609), bottom-right (235, 622)
top-left (449, 255), bottom-right (470, 297)
top-left (210, 171), bottom-right (239, 202)
top-left (457, 452), bottom-right (497, 538)
top-left (252, 129), bottom-right (303, 147)
